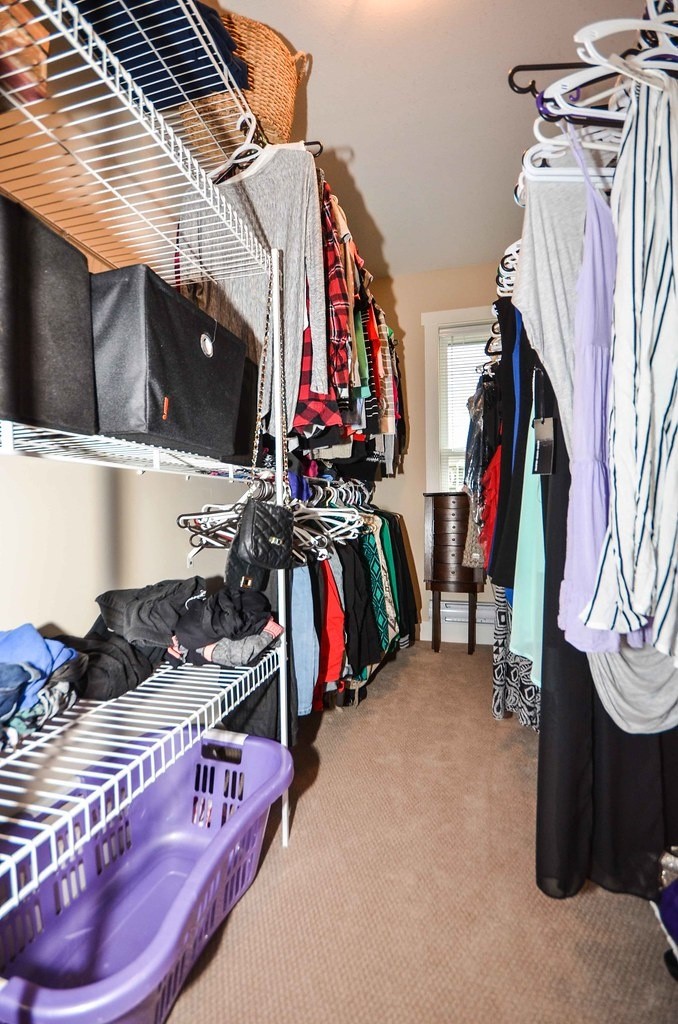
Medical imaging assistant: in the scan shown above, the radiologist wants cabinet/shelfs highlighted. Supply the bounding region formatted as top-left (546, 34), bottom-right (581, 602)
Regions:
top-left (0, 0), bottom-right (299, 921)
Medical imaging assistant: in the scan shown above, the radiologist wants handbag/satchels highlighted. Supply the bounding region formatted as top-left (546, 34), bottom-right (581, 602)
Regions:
top-left (224, 498), bottom-right (294, 592)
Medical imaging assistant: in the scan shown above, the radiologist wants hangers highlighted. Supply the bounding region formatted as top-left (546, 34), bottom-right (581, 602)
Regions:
top-left (203, 112), bottom-right (322, 187)
top-left (474, 0), bottom-right (678, 377)
top-left (176, 477), bottom-right (401, 566)
